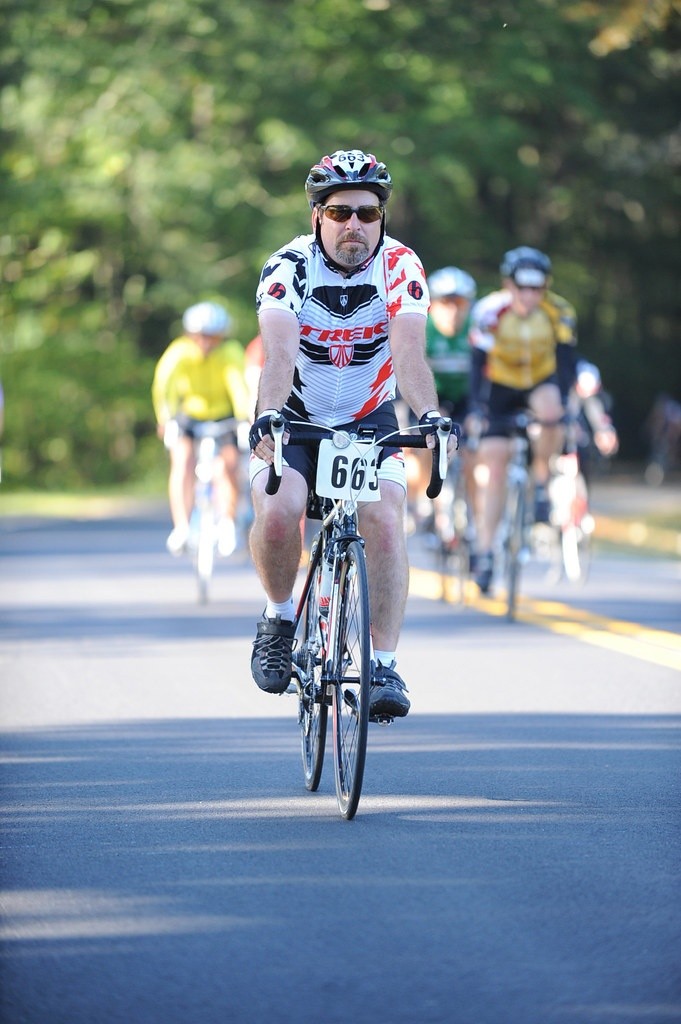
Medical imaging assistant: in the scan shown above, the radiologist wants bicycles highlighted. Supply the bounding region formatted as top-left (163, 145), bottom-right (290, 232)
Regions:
top-left (187, 407), bottom-right (588, 623)
top-left (266, 411), bottom-right (453, 822)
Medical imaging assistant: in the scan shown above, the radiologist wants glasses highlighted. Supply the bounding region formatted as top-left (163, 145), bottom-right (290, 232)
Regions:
top-left (321, 204), bottom-right (383, 223)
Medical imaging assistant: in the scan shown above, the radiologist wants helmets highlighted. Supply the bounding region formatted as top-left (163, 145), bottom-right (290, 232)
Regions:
top-left (500, 246), bottom-right (552, 289)
top-left (305, 149), bottom-right (393, 209)
top-left (427, 266), bottom-right (478, 299)
top-left (182, 301), bottom-right (230, 336)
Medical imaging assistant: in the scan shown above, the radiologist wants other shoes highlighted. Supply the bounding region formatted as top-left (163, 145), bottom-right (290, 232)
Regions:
top-left (474, 549), bottom-right (496, 592)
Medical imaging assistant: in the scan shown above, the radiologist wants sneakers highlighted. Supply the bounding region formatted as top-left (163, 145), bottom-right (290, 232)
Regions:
top-left (369, 658), bottom-right (410, 717)
top-left (251, 605), bottom-right (298, 694)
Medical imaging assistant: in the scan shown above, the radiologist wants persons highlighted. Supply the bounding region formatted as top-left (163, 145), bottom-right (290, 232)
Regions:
top-left (250, 152), bottom-right (458, 717)
top-left (153, 245), bottom-right (617, 595)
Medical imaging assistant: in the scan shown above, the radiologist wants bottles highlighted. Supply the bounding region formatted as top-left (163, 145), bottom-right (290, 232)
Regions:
top-left (319, 553), bottom-right (334, 616)
